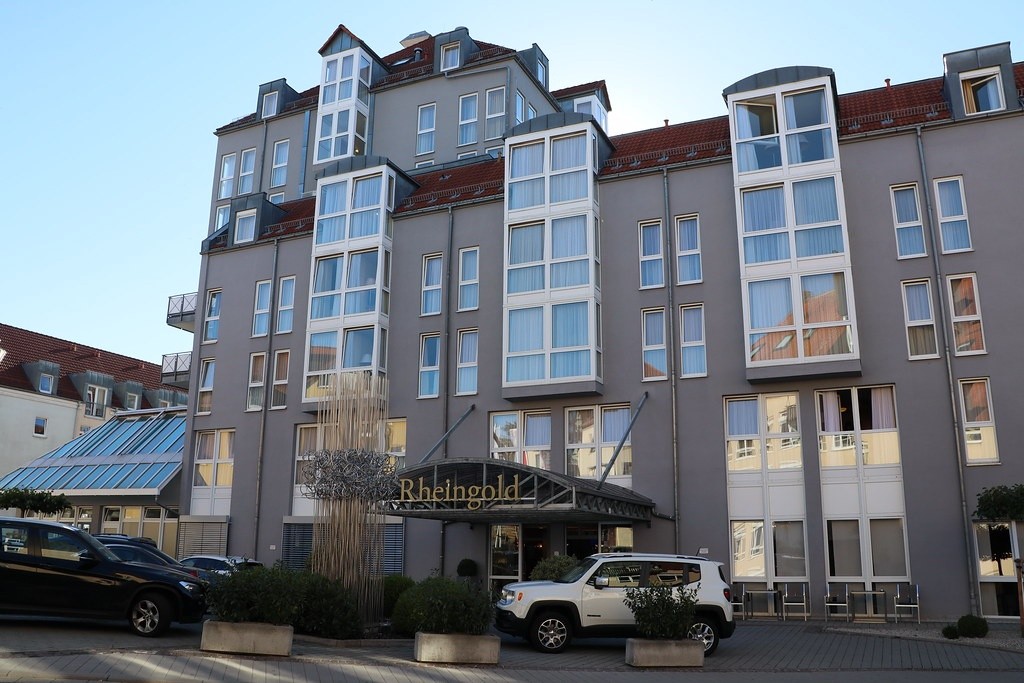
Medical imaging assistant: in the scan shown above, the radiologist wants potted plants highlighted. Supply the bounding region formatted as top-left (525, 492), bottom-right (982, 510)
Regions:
top-left (201, 556), bottom-right (308, 656)
top-left (623, 580), bottom-right (705, 667)
top-left (411, 568), bottom-right (501, 663)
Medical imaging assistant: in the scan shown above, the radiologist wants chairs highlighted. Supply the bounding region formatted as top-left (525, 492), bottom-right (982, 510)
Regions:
top-left (894, 584), bottom-right (920, 624)
top-left (782, 584), bottom-right (806, 622)
top-left (824, 584), bottom-right (849, 623)
top-left (728, 583), bottom-right (748, 620)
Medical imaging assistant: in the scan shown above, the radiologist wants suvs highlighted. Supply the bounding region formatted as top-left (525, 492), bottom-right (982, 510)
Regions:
top-left (494, 552), bottom-right (737, 657)
top-left (0, 516), bottom-right (208, 637)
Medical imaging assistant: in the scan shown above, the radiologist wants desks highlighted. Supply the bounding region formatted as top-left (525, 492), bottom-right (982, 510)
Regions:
top-left (746, 590), bottom-right (782, 619)
top-left (850, 591), bottom-right (887, 623)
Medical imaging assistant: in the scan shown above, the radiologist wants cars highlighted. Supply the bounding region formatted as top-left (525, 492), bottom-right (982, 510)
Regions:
top-left (80, 533), bottom-right (224, 606)
top-left (179, 555), bottom-right (264, 579)
